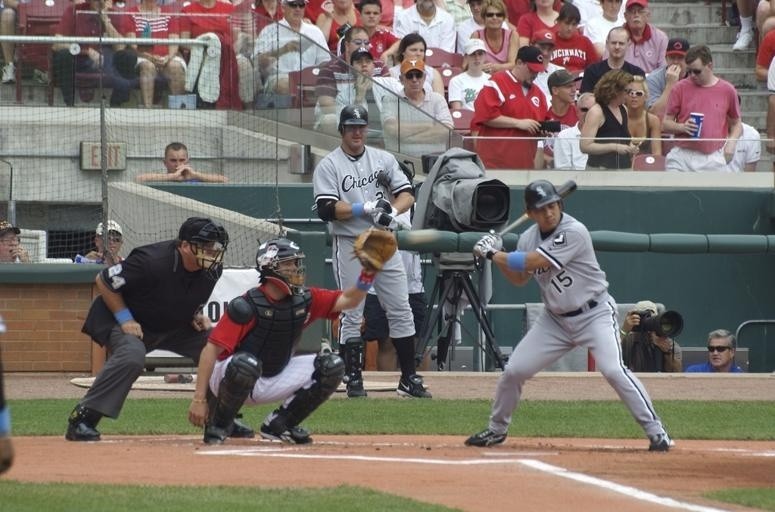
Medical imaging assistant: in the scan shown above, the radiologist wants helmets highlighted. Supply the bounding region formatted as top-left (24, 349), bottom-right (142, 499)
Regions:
top-left (339, 104), bottom-right (368, 135)
top-left (256, 238), bottom-right (308, 297)
top-left (524, 180), bottom-right (565, 212)
top-left (178, 217), bottom-right (229, 282)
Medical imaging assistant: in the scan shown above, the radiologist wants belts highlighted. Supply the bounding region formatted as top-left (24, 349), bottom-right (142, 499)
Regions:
top-left (560, 301), bottom-right (598, 317)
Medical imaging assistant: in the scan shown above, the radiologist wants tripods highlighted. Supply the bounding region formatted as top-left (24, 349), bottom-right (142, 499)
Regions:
top-left (416, 272), bottom-right (506, 373)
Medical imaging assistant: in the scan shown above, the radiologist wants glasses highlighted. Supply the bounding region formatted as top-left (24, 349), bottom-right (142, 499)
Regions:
top-left (0, 238), bottom-right (20, 245)
top-left (685, 68), bottom-right (702, 74)
top-left (353, 40), bottom-right (370, 45)
top-left (287, 2), bottom-right (306, 8)
top-left (486, 13), bottom-right (503, 18)
top-left (580, 108), bottom-right (588, 112)
top-left (622, 89), bottom-right (632, 94)
top-left (630, 90), bottom-right (645, 97)
top-left (406, 73), bottom-right (423, 79)
top-left (708, 346), bottom-right (732, 352)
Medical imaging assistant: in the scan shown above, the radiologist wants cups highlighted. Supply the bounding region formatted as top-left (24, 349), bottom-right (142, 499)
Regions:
top-left (689, 112), bottom-right (704, 137)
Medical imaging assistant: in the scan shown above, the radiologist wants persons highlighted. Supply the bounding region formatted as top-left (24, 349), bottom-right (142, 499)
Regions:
top-left (0, 0), bottom-right (254, 108)
top-left (686, 329), bottom-right (743, 372)
top-left (65, 217), bottom-right (254, 440)
top-left (463, 180), bottom-right (673, 452)
top-left (1, 220), bottom-right (28, 264)
top-left (619, 301), bottom-right (683, 372)
top-left (136, 142), bottom-right (228, 183)
top-left (256, 0), bottom-right (775, 170)
top-left (74, 220), bottom-right (123, 264)
top-left (363, 162), bottom-right (430, 370)
top-left (187, 227), bottom-right (396, 447)
top-left (312, 105), bottom-right (432, 397)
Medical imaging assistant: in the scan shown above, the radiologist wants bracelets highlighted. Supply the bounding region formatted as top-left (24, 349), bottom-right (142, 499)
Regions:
top-left (664, 346), bottom-right (673, 355)
top-left (192, 397), bottom-right (206, 403)
top-left (358, 272), bottom-right (374, 292)
top-left (115, 310), bottom-right (132, 325)
top-left (620, 330), bottom-right (625, 334)
top-left (352, 203), bottom-right (362, 215)
top-left (507, 251), bottom-right (526, 274)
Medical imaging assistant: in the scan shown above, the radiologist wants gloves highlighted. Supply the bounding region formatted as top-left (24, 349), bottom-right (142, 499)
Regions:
top-left (371, 212), bottom-right (398, 229)
top-left (364, 198), bottom-right (393, 215)
top-left (474, 228), bottom-right (504, 261)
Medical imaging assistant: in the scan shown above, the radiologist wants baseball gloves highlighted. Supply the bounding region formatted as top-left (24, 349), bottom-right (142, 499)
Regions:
top-left (352, 230), bottom-right (396, 273)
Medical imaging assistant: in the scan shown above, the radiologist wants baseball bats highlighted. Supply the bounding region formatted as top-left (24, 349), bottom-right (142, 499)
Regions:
top-left (498, 180), bottom-right (577, 238)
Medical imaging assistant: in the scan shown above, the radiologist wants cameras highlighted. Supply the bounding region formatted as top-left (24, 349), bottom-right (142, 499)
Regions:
top-left (630, 310), bottom-right (684, 338)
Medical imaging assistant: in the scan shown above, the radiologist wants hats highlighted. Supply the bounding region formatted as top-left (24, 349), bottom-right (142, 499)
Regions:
top-left (0, 220), bottom-right (20, 237)
top-left (96, 219), bottom-right (122, 235)
top-left (461, 39), bottom-right (488, 57)
top-left (625, 1), bottom-right (648, 8)
top-left (400, 57), bottom-right (425, 75)
top-left (351, 48), bottom-right (373, 64)
top-left (532, 29), bottom-right (556, 47)
top-left (634, 301), bottom-right (658, 318)
top-left (666, 38), bottom-right (689, 56)
top-left (517, 46), bottom-right (548, 73)
top-left (548, 70), bottom-right (583, 86)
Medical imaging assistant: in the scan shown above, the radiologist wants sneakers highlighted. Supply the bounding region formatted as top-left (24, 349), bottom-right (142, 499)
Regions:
top-left (204, 425), bottom-right (224, 444)
top-left (397, 374), bottom-right (432, 397)
top-left (650, 433), bottom-right (671, 450)
top-left (732, 29), bottom-right (755, 51)
top-left (347, 379), bottom-right (367, 396)
top-left (33, 67), bottom-right (49, 83)
top-left (66, 422), bottom-right (101, 441)
top-left (465, 428), bottom-right (507, 446)
top-left (231, 420), bottom-right (254, 438)
top-left (2, 62), bottom-right (17, 84)
top-left (260, 422), bottom-right (308, 443)
